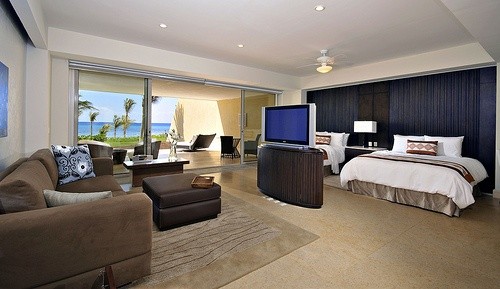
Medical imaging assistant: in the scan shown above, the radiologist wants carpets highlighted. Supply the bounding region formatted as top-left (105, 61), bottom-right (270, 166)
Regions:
top-left (126, 187), bottom-right (320, 289)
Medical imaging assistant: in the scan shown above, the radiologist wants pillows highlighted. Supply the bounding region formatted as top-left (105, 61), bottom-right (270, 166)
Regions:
top-left (51, 144), bottom-right (95, 185)
top-left (391, 135), bottom-right (464, 157)
top-left (44, 190), bottom-right (112, 207)
top-left (316, 131), bottom-right (350, 146)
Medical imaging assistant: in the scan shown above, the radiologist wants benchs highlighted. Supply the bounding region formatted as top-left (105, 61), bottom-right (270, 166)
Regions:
top-left (143, 173), bottom-right (221, 231)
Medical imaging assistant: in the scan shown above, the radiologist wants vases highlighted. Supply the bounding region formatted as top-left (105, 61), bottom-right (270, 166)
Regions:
top-left (170, 146), bottom-right (177, 158)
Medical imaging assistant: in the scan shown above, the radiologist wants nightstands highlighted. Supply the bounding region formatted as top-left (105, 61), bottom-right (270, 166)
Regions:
top-left (339, 146), bottom-right (387, 173)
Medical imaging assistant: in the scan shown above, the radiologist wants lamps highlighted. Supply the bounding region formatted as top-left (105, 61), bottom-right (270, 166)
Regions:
top-left (316, 63), bottom-right (333, 74)
top-left (354, 121), bottom-right (377, 148)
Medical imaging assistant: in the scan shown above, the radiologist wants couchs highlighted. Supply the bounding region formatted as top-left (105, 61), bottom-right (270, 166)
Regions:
top-left (77, 143), bottom-right (113, 158)
top-left (0, 148), bottom-right (153, 289)
top-left (130, 141), bottom-right (161, 161)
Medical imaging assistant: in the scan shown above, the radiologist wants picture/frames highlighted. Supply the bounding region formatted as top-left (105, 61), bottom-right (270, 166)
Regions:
top-left (238, 113), bottom-right (247, 127)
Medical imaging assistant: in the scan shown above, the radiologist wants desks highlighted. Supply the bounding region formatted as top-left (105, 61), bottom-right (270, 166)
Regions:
top-left (257, 144), bottom-right (323, 209)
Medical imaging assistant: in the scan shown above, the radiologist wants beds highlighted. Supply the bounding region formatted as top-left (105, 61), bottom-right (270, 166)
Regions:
top-left (340, 135), bottom-right (489, 218)
top-left (316, 131), bottom-right (350, 177)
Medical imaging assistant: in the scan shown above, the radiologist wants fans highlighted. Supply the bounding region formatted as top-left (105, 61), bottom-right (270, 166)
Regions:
top-left (296, 50), bottom-right (352, 69)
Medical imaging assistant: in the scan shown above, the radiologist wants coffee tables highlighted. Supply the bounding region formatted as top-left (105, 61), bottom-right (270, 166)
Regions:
top-left (123, 158), bottom-right (190, 187)
top-left (113, 149), bottom-right (127, 164)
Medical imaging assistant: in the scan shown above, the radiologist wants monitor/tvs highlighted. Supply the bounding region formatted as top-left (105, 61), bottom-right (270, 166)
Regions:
top-left (262, 103), bottom-right (316, 147)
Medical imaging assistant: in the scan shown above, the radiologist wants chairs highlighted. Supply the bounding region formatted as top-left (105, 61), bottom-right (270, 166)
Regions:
top-left (244, 134), bottom-right (261, 159)
top-left (220, 136), bottom-right (236, 159)
